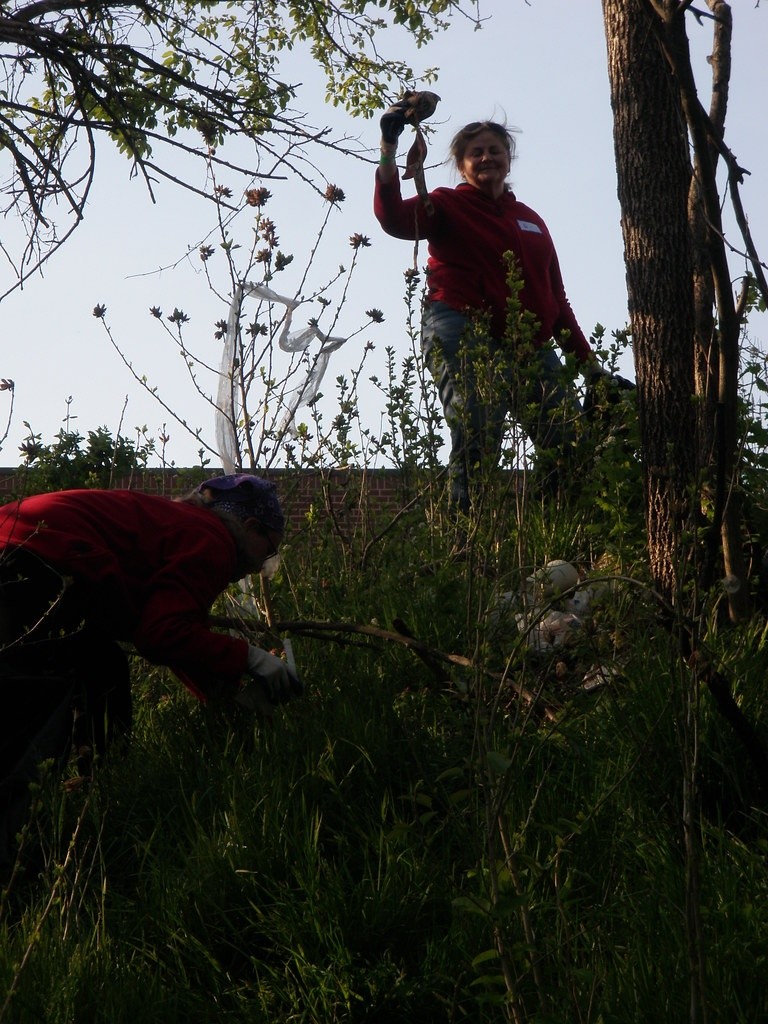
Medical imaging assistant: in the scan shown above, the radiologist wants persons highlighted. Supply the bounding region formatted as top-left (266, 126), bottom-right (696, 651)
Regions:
top-left (373, 98), bottom-right (618, 571)
top-left (0, 474), bottom-right (304, 924)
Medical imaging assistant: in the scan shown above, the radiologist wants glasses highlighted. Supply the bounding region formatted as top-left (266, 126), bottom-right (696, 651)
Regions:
top-left (458, 122), bottom-right (508, 137)
top-left (252, 518), bottom-right (279, 561)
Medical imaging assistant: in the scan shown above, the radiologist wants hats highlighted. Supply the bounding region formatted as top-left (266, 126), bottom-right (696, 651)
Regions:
top-left (188, 474), bottom-right (286, 529)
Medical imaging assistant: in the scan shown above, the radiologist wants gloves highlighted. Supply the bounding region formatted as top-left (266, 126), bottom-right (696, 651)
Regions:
top-left (246, 646), bottom-right (304, 707)
top-left (380, 109), bottom-right (407, 152)
